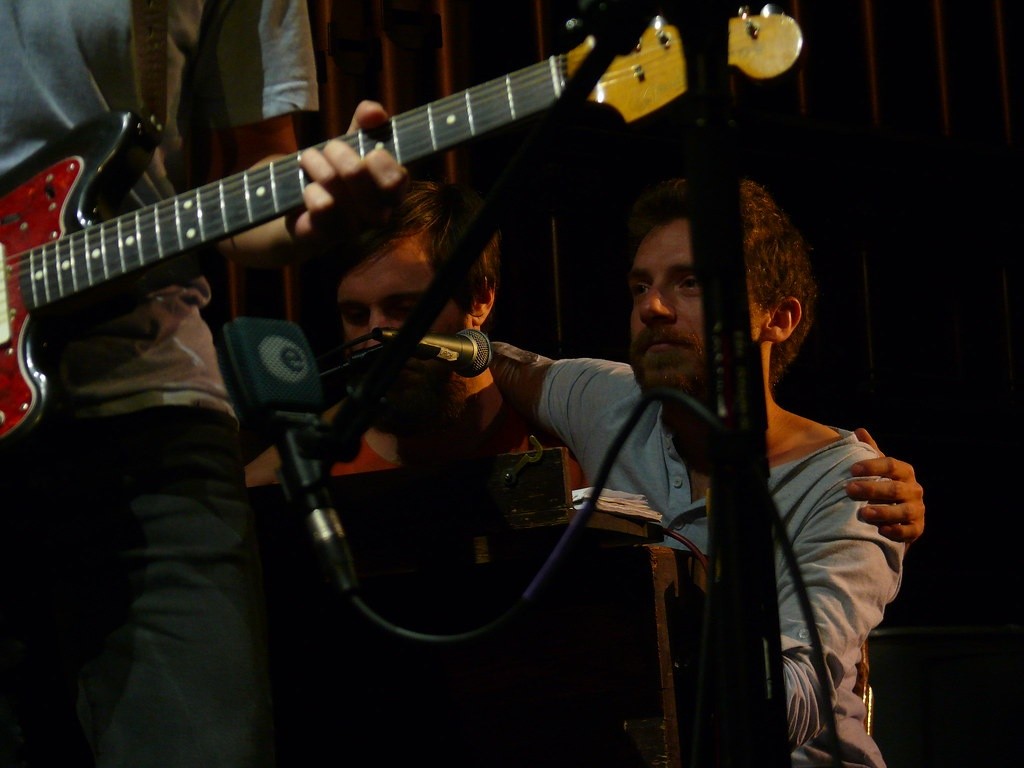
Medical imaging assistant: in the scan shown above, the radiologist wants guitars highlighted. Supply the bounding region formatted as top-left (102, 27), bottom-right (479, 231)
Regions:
top-left (0, 0), bottom-right (827, 452)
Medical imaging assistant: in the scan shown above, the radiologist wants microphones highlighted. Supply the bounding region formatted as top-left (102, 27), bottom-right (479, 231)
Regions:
top-left (220, 318), bottom-right (360, 597)
top-left (371, 327), bottom-right (493, 378)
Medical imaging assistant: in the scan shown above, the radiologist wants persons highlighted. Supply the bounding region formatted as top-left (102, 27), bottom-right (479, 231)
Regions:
top-left (244, 178), bottom-right (925, 768)
top-left (0, 0), bottom-right (407, 768)
